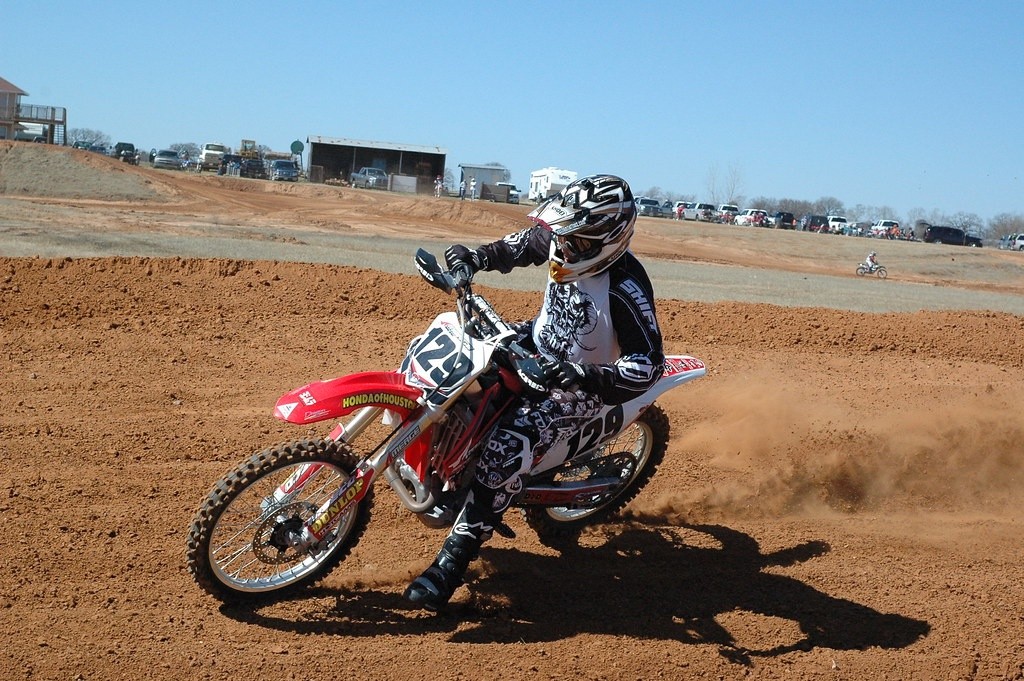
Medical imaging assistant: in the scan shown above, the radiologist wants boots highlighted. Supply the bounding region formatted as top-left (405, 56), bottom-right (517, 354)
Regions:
top-left (403, 484), bottom-right (503, 614)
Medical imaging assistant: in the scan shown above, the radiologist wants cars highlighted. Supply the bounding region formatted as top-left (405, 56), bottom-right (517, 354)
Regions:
top-left (73, 141), bottom-right (141, 166)
top-left (634, 196), bottom-right (900, 238)
top-left (149, 149), bottom-right (180, 169)
top-left (998, 232), bottom-right (1024, 251)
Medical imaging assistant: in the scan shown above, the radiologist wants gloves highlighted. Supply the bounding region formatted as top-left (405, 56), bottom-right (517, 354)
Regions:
top-left (540, 358), bottom-right (594, 394)
top-left (444, 244), bottom-right (485, 278)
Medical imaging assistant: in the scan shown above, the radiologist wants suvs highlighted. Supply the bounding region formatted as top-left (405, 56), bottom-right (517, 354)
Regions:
top-left (197, 143), bottom-right (231, 173)
top-left (222, 155), bottom-right (298, 182)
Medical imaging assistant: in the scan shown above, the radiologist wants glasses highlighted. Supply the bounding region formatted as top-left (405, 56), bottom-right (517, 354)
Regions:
top-left (557, 234), bottom-right (596, 257)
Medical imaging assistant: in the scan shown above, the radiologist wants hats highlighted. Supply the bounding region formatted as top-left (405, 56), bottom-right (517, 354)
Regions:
top-left (471, 178), bottom-right (474, 181)
top-left (217, 158), bottom-right (223, 160)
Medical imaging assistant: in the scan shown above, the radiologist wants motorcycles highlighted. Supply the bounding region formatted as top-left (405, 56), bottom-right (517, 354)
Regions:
top-left (185, 248), bottom-right (706, 604)
top-left (856, 260), bottom-right (887, 278)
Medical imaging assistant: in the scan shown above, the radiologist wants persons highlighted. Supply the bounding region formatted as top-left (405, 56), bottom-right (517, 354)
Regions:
top-left (1000, 233), bottom-right (1018, 250)
top-left (470, 178), bottom-right (477, 201)
top-left (887, 224), bottom-right (935, 242)
top-left (674, 204), bottom-right (868, 237)
top-left (537, 193), bottom-right (543, 205)
top-left (460, 180), bottom-right (468, 200)
top-left (434, 175), bottom-right (442, 197)
top-left (401, 174), bottom-right (666, 613)
top-left (217, 158), bottom-right (236, 175)
top-left (866, 251), bottom-right (878, 273)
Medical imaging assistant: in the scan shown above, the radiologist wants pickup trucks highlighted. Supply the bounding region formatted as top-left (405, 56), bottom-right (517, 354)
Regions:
top-left (350, 167), bottom-right (388, 189)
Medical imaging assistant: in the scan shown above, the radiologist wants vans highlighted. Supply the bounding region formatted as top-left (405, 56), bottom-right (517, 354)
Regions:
top-left (924, 226), bottom-right (982, 247)
top-left (496, 181), bottom-right (521, 204)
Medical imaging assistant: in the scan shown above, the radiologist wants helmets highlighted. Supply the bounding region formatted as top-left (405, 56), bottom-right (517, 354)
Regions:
top-left (527, 174), bottom-right (637, 285)
top-left (869, 251), bottom-right (876, 257)
